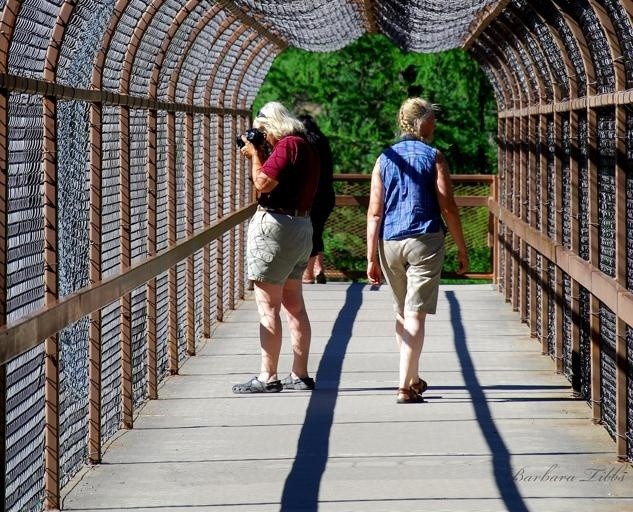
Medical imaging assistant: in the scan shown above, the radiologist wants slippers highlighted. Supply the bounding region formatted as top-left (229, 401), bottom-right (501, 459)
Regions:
top-left (302, 272), bottom-right (326, 284)
top-left (280, 373), bottom-right (315, 390)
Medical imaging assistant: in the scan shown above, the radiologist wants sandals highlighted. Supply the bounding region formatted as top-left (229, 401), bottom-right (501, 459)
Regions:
top-left (396, 387), bottom-right (423, 403)
top-left (232, 376), bottom-right (283, 393)
top-left (409, 379), bottom-right (427, 393)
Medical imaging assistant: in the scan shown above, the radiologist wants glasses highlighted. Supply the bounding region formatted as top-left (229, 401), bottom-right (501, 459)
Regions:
top-left (256, 111), bottom-right (268, 119)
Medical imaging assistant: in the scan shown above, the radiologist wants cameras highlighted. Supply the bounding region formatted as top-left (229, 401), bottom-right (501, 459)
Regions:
top-left (236, 128), bottom-right (265, 147)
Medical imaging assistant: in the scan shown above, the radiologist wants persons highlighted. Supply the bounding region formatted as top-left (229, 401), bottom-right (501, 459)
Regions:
top-left (231, 101), bottom-right (316, 395)
top-left (366, 96), bottom-right (469, 404)
top-left (294, 115), bottom-right (336, 283)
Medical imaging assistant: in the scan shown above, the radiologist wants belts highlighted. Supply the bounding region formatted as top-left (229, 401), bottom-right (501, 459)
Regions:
top-left (257, 205), bottom-right (312, 216)
